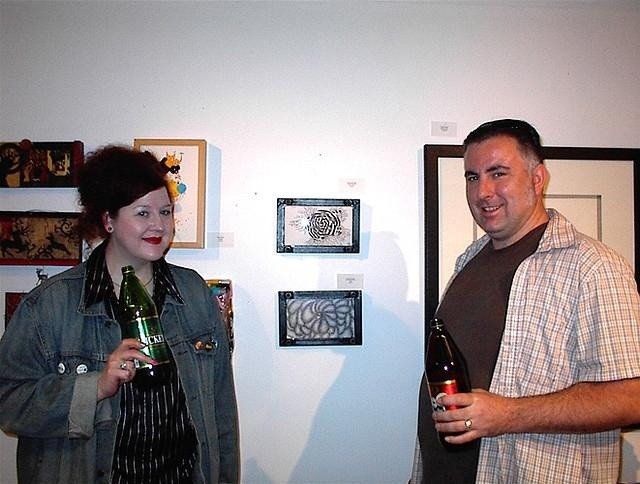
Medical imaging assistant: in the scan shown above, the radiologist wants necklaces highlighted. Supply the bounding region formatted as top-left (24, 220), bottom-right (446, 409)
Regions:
top-left (109, 267), bottom-right (156, 291)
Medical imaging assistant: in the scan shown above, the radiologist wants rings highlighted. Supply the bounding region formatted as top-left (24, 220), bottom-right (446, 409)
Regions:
top-left (463, 418), bottom-right (473, 432)
top-left (118, 359), bottom-right (128, 371)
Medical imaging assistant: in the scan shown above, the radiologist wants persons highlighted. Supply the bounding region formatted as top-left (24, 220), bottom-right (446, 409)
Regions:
top-left (0, 141), bottom-right (243, 482)
top-left (407, 116), bottom-right (638, 482)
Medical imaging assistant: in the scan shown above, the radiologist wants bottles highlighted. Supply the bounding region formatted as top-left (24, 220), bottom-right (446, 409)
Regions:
top-left (424, 318), bottom-right (480, 453)
top-left (117, 265), bottom-right (173, 382)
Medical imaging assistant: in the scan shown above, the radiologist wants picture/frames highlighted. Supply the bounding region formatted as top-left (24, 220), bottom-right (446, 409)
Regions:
top-left (272, 195), bottom-right (365, 257)
top-left (2, 289), bottom-right (29, 333)
top-left (200, 278), bottom-right (237, 350)
top-left (130, 138), bottom-right (211, 249)
top-left (1, 139), bottom-right (87, 190)
top-left (1, 208), bottom-right (85, 269)
top-left (419, 139), bottom-right (640, 349)
top-left (276, 288), bottom-right (366, 347)
